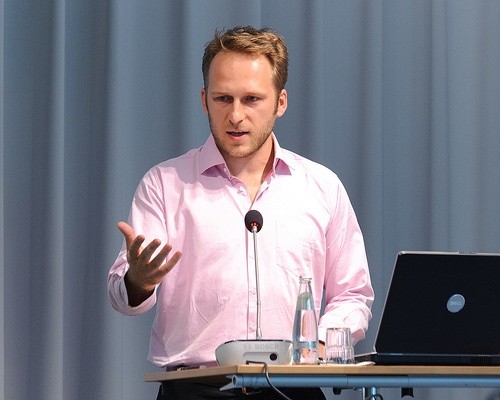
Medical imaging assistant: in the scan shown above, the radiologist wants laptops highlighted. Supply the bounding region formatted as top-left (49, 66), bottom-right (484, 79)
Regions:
top-left (352, 250), bottom-right (500, 366)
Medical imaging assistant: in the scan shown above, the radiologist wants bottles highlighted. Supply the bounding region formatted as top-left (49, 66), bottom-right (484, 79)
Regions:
top-left (291, 276), bottom-right (319, 365)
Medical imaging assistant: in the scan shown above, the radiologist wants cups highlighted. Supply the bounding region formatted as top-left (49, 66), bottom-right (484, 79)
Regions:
top-left (323, 327), bottom-right (355, 365)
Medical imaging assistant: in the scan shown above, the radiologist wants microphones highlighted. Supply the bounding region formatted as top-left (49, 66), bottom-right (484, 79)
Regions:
top-left (245, 208), bottom-right (265, 338)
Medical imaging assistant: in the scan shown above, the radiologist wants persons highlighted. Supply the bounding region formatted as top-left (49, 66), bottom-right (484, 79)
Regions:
top-left (107, 26), bottom-right (375, 400)
